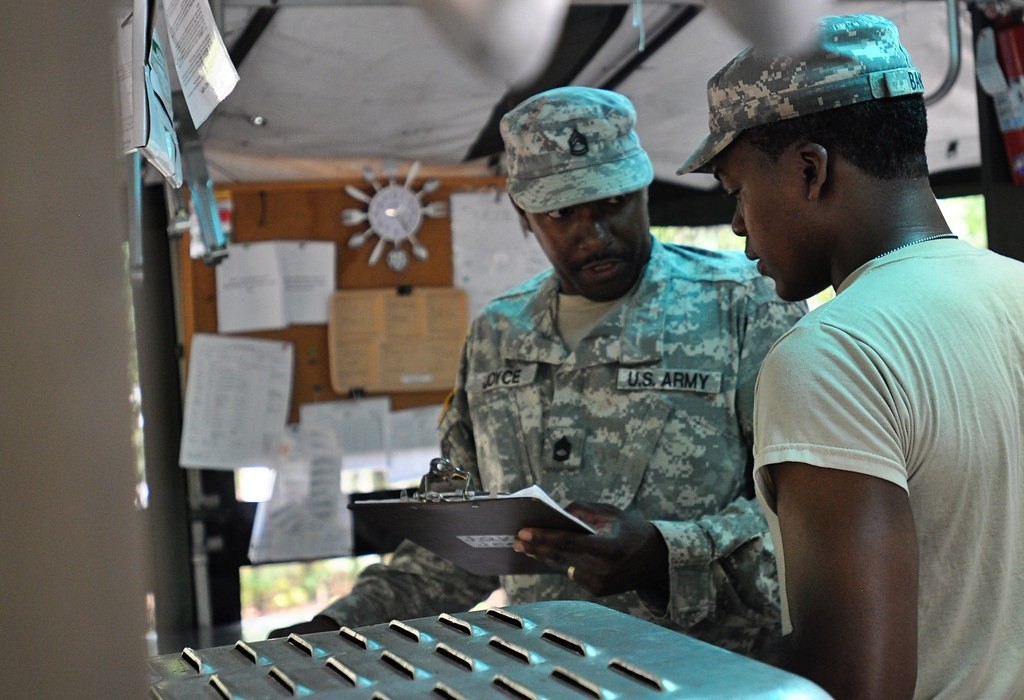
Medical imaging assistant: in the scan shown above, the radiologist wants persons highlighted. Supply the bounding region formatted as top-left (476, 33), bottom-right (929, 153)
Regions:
top-left (267, 85), bottom-right (810, 667)
top-left (674, 14), bottom-right (1024, 700)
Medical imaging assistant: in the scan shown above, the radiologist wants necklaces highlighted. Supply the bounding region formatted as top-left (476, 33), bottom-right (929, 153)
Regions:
top-left (874, 233), bottom-right (958, 259)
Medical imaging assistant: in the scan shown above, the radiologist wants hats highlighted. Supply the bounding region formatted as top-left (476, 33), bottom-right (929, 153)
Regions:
top-left (675, 13), bottom-right (923, 176)
top-left (498, 86), bottom-right (654, 213)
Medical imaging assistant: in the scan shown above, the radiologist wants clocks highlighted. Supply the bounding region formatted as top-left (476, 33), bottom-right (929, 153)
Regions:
top-left (340, 160), bottom-right (448, 269)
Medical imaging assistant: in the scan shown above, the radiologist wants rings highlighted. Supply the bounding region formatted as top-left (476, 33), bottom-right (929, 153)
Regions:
top-left (567, 566), bottom-right (576, 580)
